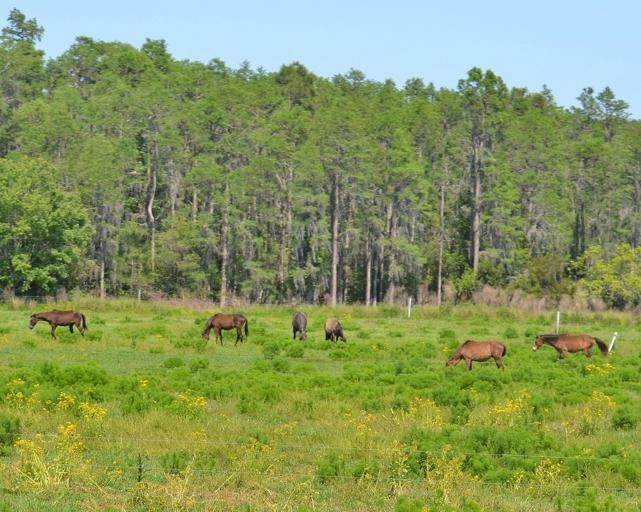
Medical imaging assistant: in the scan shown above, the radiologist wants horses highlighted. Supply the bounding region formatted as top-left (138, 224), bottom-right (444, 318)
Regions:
top-left (291, 311), bottom-right (308, 340)
top-left (201, 312), bottom-right (249, 346)
top-left (531, 333), bottom-right (609, 362)
top-left (446, 339), bottom-right (506, 372)
top-left (28, 309), bottom-right (88, 340)
top-left (323, 318), bottom-right (347, 343)
